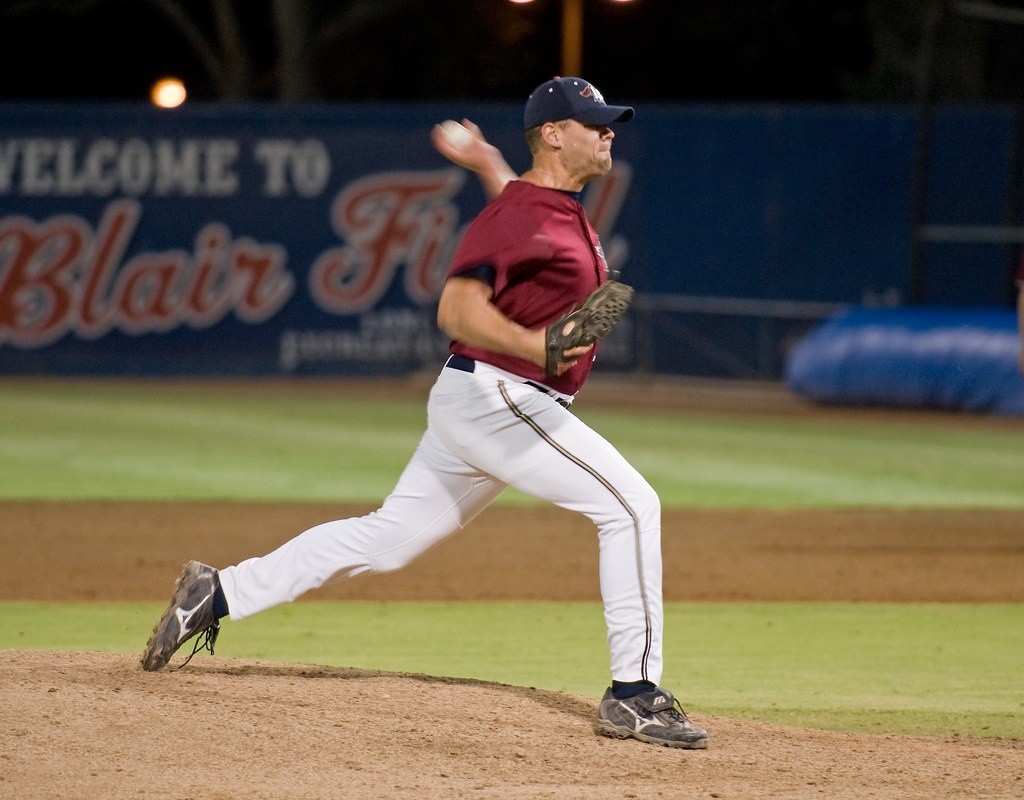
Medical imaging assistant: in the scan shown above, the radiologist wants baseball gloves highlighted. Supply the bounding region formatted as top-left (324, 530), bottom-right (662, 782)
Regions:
top-left (546, 269), bottom-right (636, 379)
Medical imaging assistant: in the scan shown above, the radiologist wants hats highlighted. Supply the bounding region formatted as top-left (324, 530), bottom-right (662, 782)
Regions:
top-left (524, 74), bottom-right (637, 130)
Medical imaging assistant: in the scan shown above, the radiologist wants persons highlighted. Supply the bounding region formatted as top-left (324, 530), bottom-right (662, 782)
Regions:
top-left (141, 77), bottom-right (710, 750)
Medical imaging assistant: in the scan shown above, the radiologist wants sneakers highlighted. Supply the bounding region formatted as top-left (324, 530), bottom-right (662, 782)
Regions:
top-left (139, 560), bottom-right (218, 672)
top-left (593, 685), bottom-right (711, 752)
